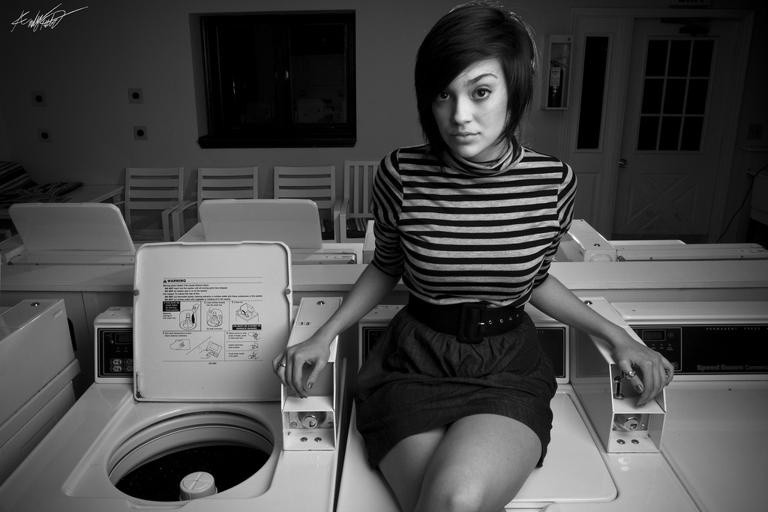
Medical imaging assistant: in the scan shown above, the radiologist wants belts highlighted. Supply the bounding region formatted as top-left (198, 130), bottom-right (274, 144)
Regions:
top-left (406, 291), bottom-right (525, 346)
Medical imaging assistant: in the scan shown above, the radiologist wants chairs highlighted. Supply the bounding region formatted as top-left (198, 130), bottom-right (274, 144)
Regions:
top-left (112, 161), bottom-right (381, 245)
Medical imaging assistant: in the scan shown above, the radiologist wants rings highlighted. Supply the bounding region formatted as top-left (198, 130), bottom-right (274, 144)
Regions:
top-left (278, 363), bottom-right (286, 367)
top-left (626, 369), bottom-right (637, 380)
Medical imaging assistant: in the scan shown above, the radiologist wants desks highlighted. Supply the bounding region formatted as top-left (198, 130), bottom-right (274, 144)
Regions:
top-left (62, 185), bottom-right (123, 207)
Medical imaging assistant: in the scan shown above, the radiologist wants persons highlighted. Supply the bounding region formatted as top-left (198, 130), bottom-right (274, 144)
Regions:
top-left (271, 0), bottom-right (677, 512)
